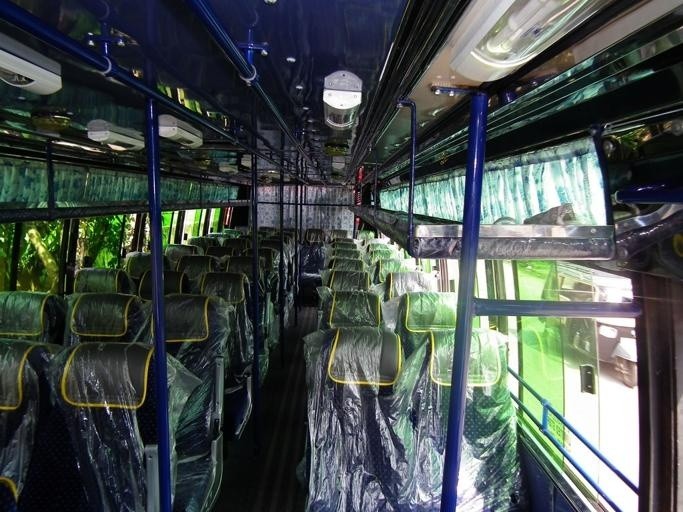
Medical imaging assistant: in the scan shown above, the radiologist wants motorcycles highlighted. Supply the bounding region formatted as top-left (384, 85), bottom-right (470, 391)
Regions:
top-left (538, 262), bottom-right (638, 388)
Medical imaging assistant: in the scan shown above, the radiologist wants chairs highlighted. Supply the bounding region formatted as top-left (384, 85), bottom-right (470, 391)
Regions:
top-left (1, 227), bottom-right (300, 512)
top-left (304, 228), bottom-right (517, 512)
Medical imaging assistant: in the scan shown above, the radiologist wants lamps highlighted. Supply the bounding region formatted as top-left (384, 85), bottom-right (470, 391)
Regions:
top-left (0, 50), bottom-right (62, 96)
top-left (158, 113), bottom-right (203, 138)
top-left (87, 131), bottom-right (144, 153)
top-left (323, 90), bottom-right (363, 132)
top-left (87, 120), bottom-right (145, 140)
top-left (450, 1), bottom-right (609, 83)
top-left (158, 127), bottom-right (203, 150)
top-left (0, 32), bottom-right (61, 75)
